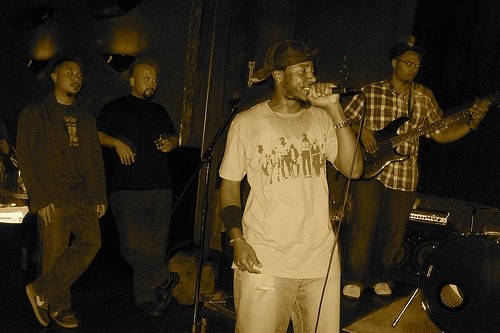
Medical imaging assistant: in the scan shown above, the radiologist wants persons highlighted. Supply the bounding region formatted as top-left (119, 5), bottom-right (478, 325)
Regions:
top-left (219, 41), bottom-right (363, 333)
top-left (341, 42), bottom-right (493, 301)
top-left (16, 57), bottom-right (108, 327)
top-left (95, 59), bottom-right (180, 317)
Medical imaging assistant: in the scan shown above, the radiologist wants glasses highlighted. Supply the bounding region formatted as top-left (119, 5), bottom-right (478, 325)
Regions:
top-left (399, 60), bottom-right (420, 69)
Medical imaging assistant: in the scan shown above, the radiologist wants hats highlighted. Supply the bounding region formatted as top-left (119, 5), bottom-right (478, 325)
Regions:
top-left (249, 40), bottom-right (314, 83)
top-left (389, 42), bottom-right (423, 60)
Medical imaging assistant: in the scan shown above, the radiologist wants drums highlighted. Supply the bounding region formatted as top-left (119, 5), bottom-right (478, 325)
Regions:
top-left (417, 230), bottom-right (500, 333)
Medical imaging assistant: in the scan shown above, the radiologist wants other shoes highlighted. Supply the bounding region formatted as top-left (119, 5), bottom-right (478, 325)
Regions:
top-left (132, 293), bottom-right (164, 316)
top-left (373, 283), bottom-right (392, 295)
top-left (156, 273), bottom-right (179, 312)
top-left (50, 310), bottom-right (78, 328)
top-left (343, 285), bottom-right (361, 300)
top-left (26, 282), bottom-right (51, 326)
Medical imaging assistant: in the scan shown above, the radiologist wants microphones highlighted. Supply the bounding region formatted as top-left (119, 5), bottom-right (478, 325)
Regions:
top-left (313, 87), bottom-right (363, 98)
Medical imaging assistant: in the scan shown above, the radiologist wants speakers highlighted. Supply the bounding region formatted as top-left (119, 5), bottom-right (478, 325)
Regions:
top-left (391, 221), bottom-right (458, 284)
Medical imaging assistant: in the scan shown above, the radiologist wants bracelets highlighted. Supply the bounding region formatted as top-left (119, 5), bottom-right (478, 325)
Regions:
top-left (229, 235), bottom-right (245, 247)
top-left (468, 120), bottom-right (478, 132)
top-left (334, 118), bottom-right (352, 130)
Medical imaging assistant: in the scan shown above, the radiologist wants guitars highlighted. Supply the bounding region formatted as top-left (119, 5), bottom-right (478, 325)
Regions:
top-left (355, 97), bottom-right (494, 180)
